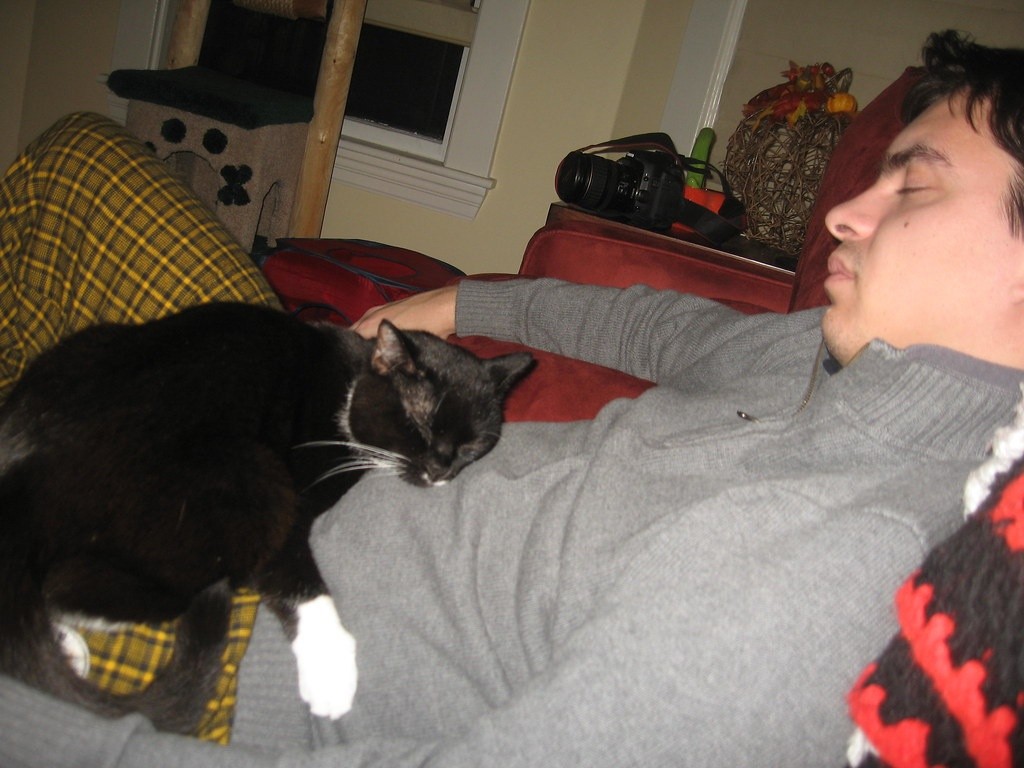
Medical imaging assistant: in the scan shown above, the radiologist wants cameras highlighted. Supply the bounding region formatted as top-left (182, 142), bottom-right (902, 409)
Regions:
top-left (555, 147), bottom-right (684, 232)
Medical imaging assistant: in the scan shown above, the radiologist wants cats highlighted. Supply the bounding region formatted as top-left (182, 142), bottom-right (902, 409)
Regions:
top-left (0, 299), bottom-right (533, 731)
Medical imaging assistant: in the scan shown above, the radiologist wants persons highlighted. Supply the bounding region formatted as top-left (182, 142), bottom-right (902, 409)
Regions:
top-left (1, 29), bottom-right (1023, 768)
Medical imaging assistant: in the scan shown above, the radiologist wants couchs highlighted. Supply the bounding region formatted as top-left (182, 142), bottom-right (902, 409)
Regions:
top-left (436, 55), bottom-right (927, 421)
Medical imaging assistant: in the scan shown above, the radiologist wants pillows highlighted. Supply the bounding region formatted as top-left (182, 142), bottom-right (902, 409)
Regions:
top-left (441, 273), bottom-right (779, 424)
